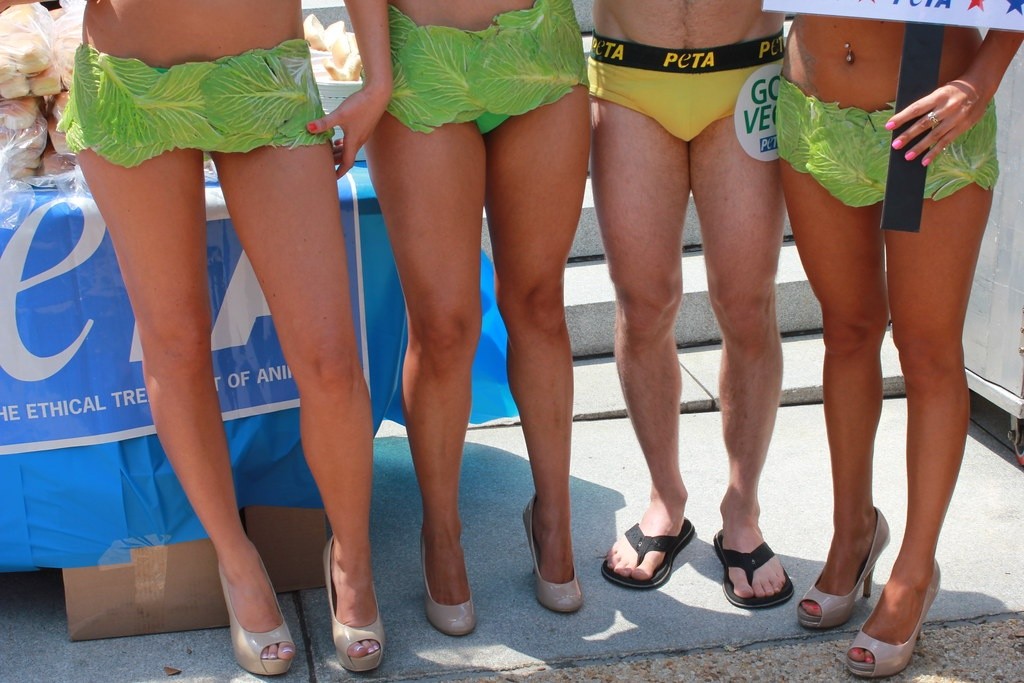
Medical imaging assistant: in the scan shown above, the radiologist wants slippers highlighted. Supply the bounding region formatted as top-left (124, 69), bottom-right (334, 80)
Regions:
top-left (714, 529), bottom-right (794, 610)
top-left (600, 517), bottom-right (695, 590)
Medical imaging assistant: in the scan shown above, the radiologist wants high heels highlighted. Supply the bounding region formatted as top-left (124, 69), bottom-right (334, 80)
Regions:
top-left (523, 495), bottom-right (585, 614)
top-left (796, 507), bottom-right (890, 632)
top-left (420, 518), bottom-right (477, 636)
top-left (218, 541), bottom-right (297, 676)
top-left (322, 536), bottom-right (385, 674)
top-left (845, 555), bottom-right (941, 678)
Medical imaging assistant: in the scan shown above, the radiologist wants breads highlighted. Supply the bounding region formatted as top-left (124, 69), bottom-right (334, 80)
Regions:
top-left (0, 0), bottom-right (87, 177)
top-left (302, 14), bottom-right (362, 83)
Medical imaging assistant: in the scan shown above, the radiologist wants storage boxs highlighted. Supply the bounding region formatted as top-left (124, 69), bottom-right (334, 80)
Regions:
top-left (244, 505), bottom-right (325, 594)
top-left (62, 540), bottom-right (231, 641)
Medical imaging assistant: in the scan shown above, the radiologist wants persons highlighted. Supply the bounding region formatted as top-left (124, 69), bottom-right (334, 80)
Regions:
top-left (777, 13), bottom-right (1024, 679)
top-left (72, 0), bottom-right (393, 676)
top-left (591, 0), bottom-right (792, 609)
top-left (361, 0), bottom-right (592, 636)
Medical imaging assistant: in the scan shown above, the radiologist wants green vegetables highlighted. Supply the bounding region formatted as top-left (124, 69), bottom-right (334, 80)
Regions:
top-left (56, 39), bottom-right (335, 168)
top-left (354, 0), bottom-right (591, 133)
top-left (775, 70), bottom-right (1000, 207)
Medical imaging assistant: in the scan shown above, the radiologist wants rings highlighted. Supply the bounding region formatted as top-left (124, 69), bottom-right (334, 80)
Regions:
top-left (928, 112), bottom-right (938, 124)
